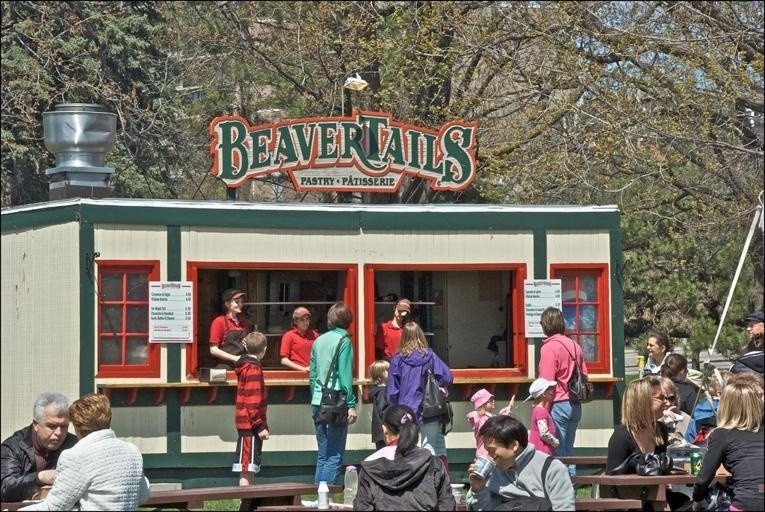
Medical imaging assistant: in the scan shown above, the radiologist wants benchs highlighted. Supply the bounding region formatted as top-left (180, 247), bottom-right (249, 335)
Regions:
top-left (254, 505), bottom-right (356, 512)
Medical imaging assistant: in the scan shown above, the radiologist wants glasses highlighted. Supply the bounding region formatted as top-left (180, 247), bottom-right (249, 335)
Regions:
top-left (652, 393), bottom-right (664, 401)
top-left (664, 396), bottom-right (675, 401)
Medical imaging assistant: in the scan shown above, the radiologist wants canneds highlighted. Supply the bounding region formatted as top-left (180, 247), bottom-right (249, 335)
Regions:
top-left (472, 456), bottom-right (495, 480)
top-left (690, 453), bottom-right (703, 475)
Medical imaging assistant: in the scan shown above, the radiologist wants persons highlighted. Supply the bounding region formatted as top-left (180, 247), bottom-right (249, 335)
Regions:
top-left (232, 332), bottom-right (270, 486)
top-left (351, 299), bottom-right (457, 511)
top-left (465, 307), bottom-right (588, 512)
top-left (308, 302), bottom-right (358, 485)
top-left (0, 392), bottom-right (79, 502)
top-left (279, 307), bottom-right (320, 371)
top-left (16, 392), bottom-right (151, 511)
top-left (209, 288), bottom-right (250, 370)
top-left (605, 310), bottom-right (764, 512)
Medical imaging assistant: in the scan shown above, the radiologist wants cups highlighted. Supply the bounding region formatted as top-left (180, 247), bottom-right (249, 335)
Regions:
top-left (38, 484), bottom-right (53, 500)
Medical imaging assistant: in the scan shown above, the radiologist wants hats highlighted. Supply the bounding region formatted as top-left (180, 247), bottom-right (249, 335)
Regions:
top-left (293, 307), bottom-right (312, 320)
top-left (471, 388), bottom-right (495, 410)
top-left (528, 377), bottom-right (557, 399)
top-left (745, 311), bottom-right (764, 322)
top-left (395, 297), bottom-right (412, 315)
top-left (221, 288), bottom-right (247, 303)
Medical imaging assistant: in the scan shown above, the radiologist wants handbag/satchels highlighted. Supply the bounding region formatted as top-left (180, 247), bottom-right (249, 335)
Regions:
top-left (704, 480), bottom-right (734, 508)
top-left (312, 385), bottom-right (348, 425)
top-left (420, 357), bottom-right (448, 418)
top-left (633, 451), bottom-right (674, 475)
top-left (591, 483), bottom-right (667, 502)
top-left (492, 497), bottom-right (556, 511)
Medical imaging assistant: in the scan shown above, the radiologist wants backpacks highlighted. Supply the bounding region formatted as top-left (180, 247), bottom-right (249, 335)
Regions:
top-left (550, 337), bottom-right (594, 403)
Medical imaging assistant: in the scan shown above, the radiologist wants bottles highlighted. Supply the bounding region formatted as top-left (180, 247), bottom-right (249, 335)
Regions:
top-left (569, 464), bottom-right (576, 476)
top-left (638, 356), bottom-right (645, 378)
top-left (452, 484), bottom-right (464, 504)
top-left (343, 466), bottom-right (358, 507)
top-left (317, 481), bottom-right (329, 510)
